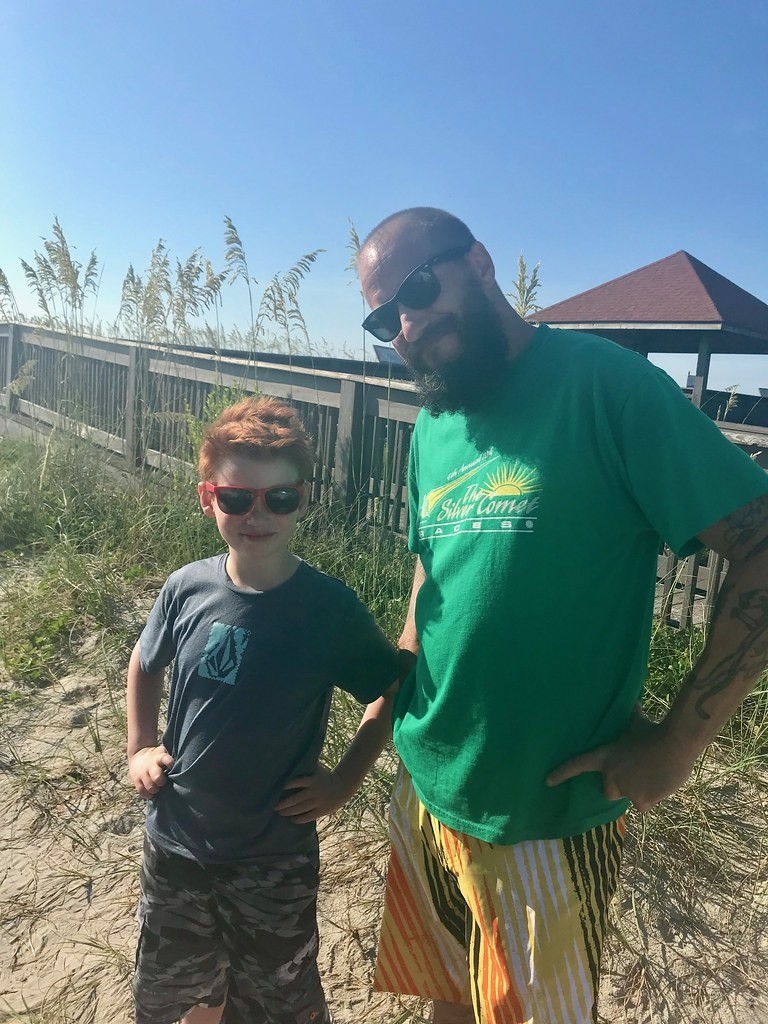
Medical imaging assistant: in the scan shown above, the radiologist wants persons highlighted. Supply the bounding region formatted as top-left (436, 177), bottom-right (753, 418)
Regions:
top-left (359, 206), bottom-right (768, 1023)
top-left (126, 396), bottom-right (401, 1024)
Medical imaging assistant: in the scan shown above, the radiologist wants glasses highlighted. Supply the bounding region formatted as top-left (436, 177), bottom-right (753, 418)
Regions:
top-left (362, 246), bottom-right (470, 343)
top-left (206, 480), bottom-right (304, 518)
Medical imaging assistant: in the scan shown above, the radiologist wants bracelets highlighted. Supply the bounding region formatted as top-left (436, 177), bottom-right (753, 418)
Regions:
top-left (400, 648), bottom-right (417, 662)
